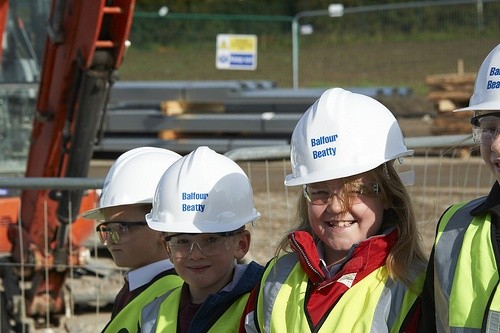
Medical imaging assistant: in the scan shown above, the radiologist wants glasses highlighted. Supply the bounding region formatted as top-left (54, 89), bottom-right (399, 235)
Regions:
top-left (471, 111), bottom-right (500, 143)
top-left (303, 182), bottom-right (379, 204)
top-left (162, 225), bottom-right (246, 257)
top-left (96, 221), bottom-right (147, 245)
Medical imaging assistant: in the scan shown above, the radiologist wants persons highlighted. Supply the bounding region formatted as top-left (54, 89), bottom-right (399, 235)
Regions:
top-left (82, 147), bottom-right (183, 320)
top-left (103, 146), bottom-right (265, 333)
top-left (239, 88), bottom-right (429, 333)
top-left (421, 43), bottom-right (500, 333)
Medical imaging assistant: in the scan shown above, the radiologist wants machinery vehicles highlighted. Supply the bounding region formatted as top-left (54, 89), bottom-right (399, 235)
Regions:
top-left (0, 0), bottom-right (150, 333)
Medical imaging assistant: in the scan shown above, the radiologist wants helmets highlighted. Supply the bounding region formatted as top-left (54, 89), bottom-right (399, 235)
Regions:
top-left (145, 146), bottom-right (261, 232)
top-left (452, 42), bottom-right (500, 111)
top-left (284, 87), bottom-right (413, 186)
top-left (80, 146), bottom-right (183, 219)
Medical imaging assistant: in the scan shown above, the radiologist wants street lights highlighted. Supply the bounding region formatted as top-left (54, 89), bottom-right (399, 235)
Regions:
top-left (291, 4), bottom-right (344, 90)
